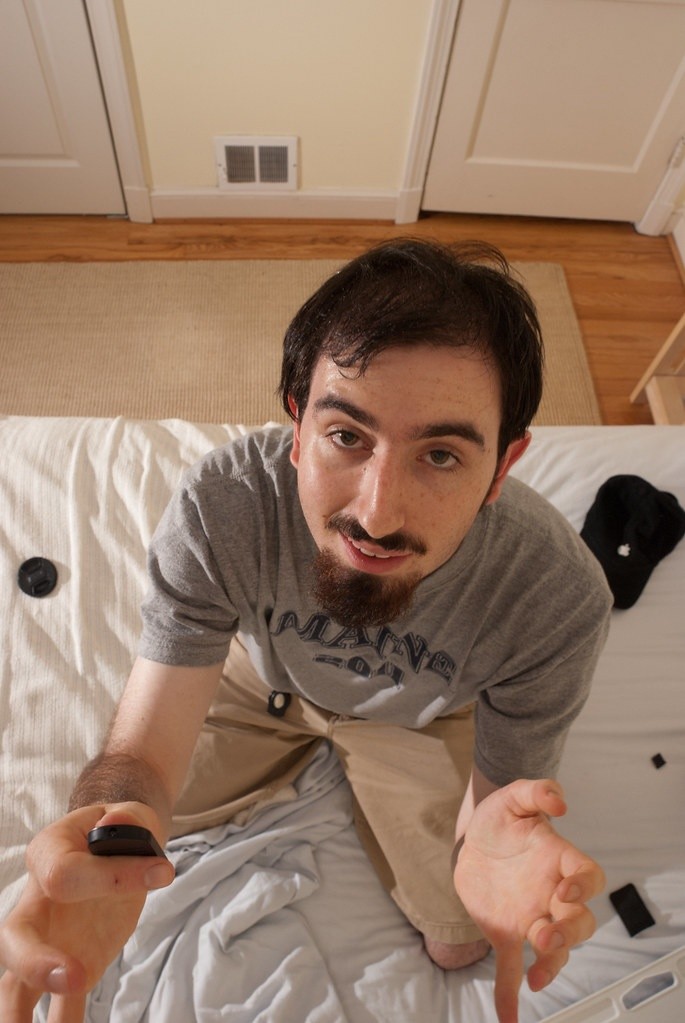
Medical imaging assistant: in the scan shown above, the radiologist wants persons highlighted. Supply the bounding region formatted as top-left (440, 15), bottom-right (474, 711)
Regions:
top-left (0, 235), bottom-right (617, 1022)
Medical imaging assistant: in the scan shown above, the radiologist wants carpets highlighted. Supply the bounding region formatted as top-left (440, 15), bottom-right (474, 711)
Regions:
top-left (2, 256), bottom-right (603, 425)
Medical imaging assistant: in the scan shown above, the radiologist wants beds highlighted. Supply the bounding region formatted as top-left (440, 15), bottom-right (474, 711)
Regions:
top-left (0, 421), bottom-right (685, 1022)
